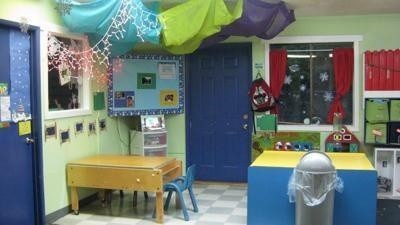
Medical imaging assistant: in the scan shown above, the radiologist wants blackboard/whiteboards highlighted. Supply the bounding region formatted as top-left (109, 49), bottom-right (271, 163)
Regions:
top-left (108, 54), bottom-right (185, 117)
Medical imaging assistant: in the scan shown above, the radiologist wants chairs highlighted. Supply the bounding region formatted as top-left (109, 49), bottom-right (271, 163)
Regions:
top-left (150, 163), bottom-right (202, 223)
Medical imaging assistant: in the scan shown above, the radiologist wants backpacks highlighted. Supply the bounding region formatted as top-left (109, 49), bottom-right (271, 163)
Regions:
top-left (246, 71), bottom-right (279, 113)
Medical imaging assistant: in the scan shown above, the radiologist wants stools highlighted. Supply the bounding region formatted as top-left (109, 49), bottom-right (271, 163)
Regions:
top-left (104, 186), bottom-right (149, 209)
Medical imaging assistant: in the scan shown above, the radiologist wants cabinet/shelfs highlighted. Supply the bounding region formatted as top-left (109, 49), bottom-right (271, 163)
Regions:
top-left (127, 128), bottom-right (169, 157)
top-left (360, 52), bottom-right (400, 146)
top-left (372, 146), bottom-right (400, 201)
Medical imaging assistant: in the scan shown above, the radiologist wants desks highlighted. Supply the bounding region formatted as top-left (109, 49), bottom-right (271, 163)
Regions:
top-left (64, 152), bottom-right (185, 224)
top-left (244, 147), bottom-right (380, 224)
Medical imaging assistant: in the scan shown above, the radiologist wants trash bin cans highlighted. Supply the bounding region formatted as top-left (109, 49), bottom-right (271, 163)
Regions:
top-left (295, 151), bottom-right (336, 225)
